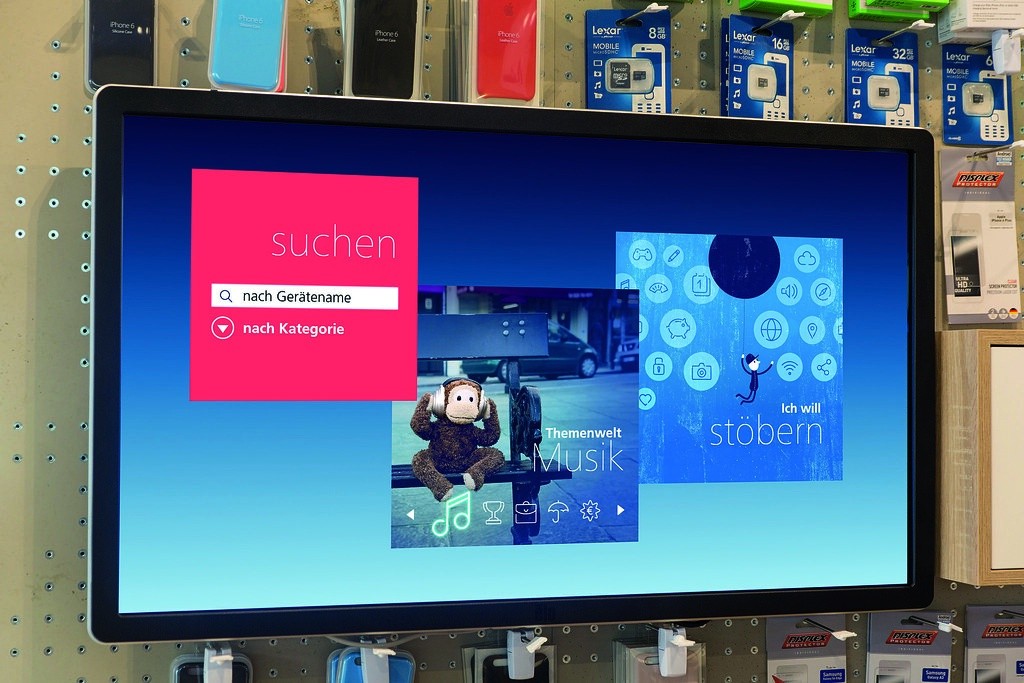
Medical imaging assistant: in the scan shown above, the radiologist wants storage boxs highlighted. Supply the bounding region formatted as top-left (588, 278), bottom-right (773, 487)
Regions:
top-left (937, 1), bottom-right (1024, 45)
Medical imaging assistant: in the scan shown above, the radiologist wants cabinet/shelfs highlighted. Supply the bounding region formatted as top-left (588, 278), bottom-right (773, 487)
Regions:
top-left (940, 329), bottom-right (1023, 585)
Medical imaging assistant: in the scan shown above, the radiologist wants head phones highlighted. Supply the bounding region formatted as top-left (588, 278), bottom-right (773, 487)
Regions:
top-left (432, 377), bottom-right (487, 422)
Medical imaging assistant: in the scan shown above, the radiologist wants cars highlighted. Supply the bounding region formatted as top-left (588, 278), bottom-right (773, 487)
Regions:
top-left (613, 336), bottom-right (639, 371)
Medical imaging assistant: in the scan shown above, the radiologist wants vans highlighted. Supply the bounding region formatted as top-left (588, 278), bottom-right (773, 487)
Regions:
top-left (462, 320), bottom-right (598, 380)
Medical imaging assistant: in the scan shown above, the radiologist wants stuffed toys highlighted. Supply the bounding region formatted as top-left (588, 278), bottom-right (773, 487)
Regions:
top-left (410, 380), bottom-right (505, 502)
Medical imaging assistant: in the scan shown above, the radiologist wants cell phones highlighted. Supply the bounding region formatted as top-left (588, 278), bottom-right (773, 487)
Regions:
top-left (632, 44), bottom-right (1010, 303)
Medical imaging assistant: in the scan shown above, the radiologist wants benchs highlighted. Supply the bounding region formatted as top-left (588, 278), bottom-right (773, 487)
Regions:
top-left (391, 313), bottom-right (572, 545)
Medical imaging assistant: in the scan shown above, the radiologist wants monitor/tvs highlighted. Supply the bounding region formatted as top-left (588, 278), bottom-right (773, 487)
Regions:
top-left (85, 83), bottom-right (934, 647)
